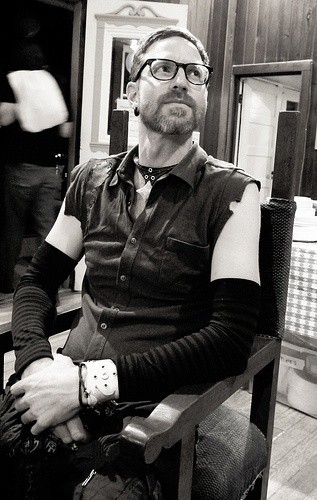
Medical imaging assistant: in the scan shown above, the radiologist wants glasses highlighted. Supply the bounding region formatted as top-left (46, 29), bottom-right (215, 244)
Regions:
top-left (132, 54), bottom-right (213, 89)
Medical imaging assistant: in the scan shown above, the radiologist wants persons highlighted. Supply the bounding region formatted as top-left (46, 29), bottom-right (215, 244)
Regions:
top-left (0, 43), bottom-right (77, 307)
top-left (1, 24), bottom-right (264, 500)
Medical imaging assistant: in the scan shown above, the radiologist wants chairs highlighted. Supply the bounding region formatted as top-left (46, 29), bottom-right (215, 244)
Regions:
top-left (0, 197), bottom-right (297, 500)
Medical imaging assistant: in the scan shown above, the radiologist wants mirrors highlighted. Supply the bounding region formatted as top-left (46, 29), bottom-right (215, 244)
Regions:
top-left (230, 59), bottom-right (312, 199)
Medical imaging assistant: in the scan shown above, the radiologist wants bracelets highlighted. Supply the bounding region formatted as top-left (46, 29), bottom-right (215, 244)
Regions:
top-left (77, 363), bottom-right (99, 410)
